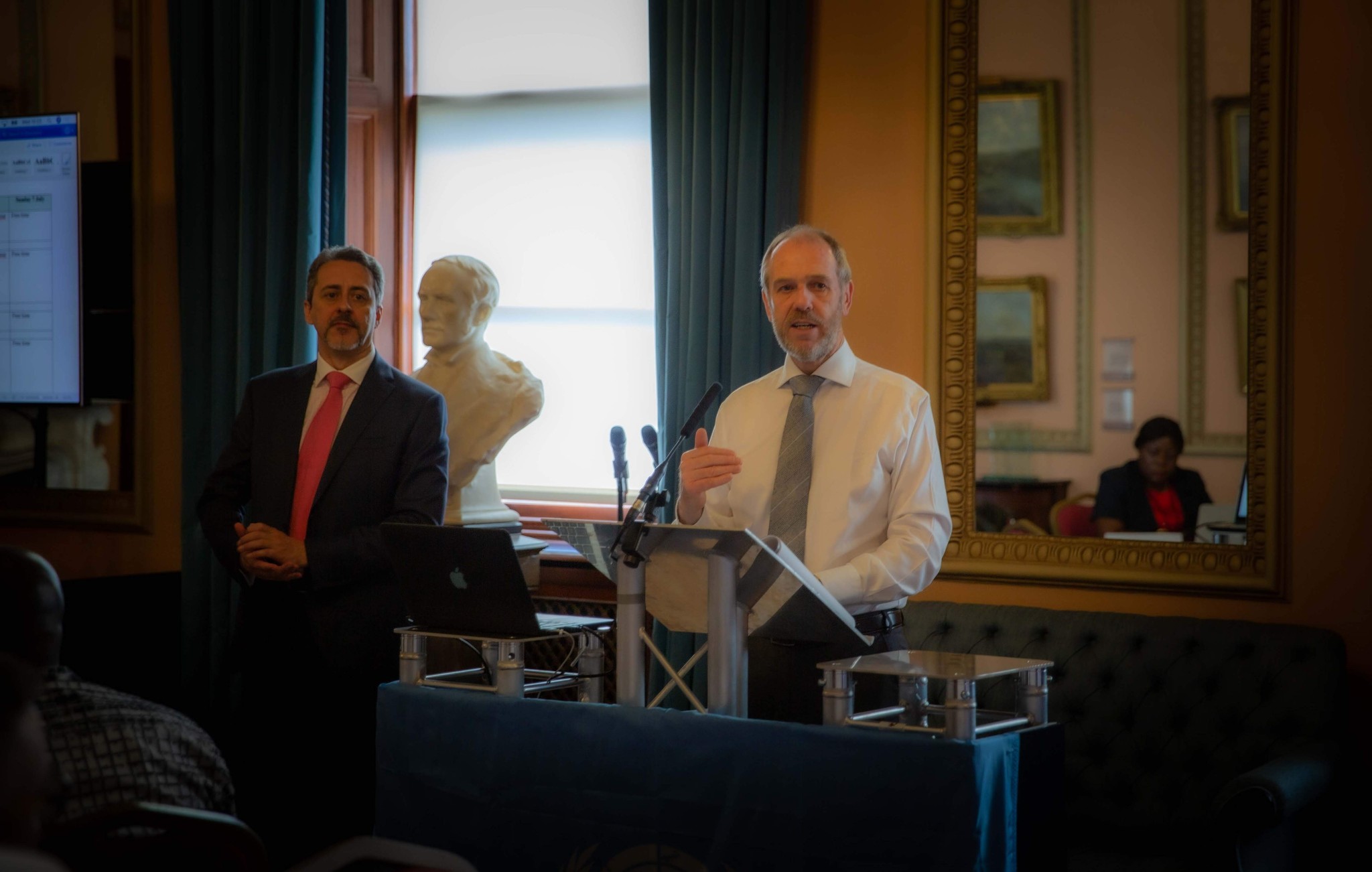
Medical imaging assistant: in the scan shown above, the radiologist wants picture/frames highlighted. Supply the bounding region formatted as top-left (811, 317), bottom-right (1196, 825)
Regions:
top-left (973, 274), bottom-right (1053, 402)
top-left (975, 75), bottom-right (1064, 235)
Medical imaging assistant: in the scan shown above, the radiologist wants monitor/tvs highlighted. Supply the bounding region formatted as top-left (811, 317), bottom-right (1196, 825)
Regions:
top-left (0, 110), bottom-right (85, 407)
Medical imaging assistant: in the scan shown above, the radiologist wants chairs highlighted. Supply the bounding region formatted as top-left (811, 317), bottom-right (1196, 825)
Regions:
top-left (1048, 490), bottom-right (1103, 538)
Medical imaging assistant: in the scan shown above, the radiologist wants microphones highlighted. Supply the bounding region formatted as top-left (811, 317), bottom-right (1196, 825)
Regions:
top-left (623, 381), bottom-right (723, 529)
top-left (610, 426), bottom-right (629, 480)
top-left (640, 425), bottom-right (658, 465)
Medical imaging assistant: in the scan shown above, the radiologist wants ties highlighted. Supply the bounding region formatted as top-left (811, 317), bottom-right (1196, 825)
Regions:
top-left (766, 373), bottom-right (825, 565)
top-left (292, 371), bottom-right (352, 543)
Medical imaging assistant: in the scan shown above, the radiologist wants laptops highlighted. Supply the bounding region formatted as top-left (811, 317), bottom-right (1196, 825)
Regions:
top-left (1206, 468), bottom-right (1249, 532)
top-left (376, 522), bottom-right (616, 638)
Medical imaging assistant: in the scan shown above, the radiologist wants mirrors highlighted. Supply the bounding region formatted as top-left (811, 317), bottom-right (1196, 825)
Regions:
top-left (1211, 93), bottom-right (1250, 231)
top-left (1234, 279), bottom-right (1247, 395)
top-left (915, 0), bottom-right (1299, 603)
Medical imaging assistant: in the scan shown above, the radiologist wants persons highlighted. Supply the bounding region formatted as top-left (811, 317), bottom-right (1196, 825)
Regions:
top-left (407, 255), bottom-right (544, 492)
top-left (1088, 416), bottom-right (1214, 543)
top-left (196, 244), bottom-right (449, 584)
top-left (672, 224), bottom-right (954, 725)
top-left (0, 550), bottom-right (241, 872)
